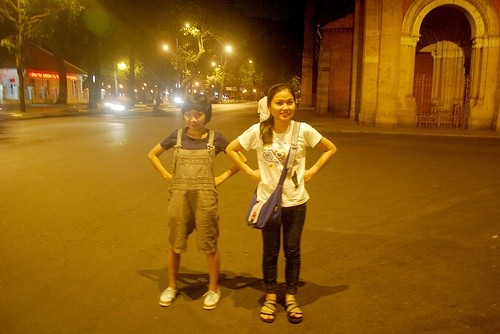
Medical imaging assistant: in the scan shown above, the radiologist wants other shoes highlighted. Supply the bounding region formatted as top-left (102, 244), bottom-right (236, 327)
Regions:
top-left (202, 286), bottom-right (221, 309)
top-left (159, 287), bottom-right (178, 306)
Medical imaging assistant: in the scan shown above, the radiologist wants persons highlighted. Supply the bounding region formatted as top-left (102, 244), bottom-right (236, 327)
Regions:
top-left (225, 83), bottom-right (338, 324)
top-left (147, 92), bottom-right (248, 310)
top-left (257, 89), bottom-right (270, 123)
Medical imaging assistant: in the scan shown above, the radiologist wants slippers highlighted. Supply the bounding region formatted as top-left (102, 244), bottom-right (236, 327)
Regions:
top-left (260, 299), bottom-right (277, 323)
top-left (285, 300), bottom-right (303, 323)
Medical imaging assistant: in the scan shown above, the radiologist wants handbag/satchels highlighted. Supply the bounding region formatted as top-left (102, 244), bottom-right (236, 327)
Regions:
top-left (245, 185), bottom-right (282, 229)
